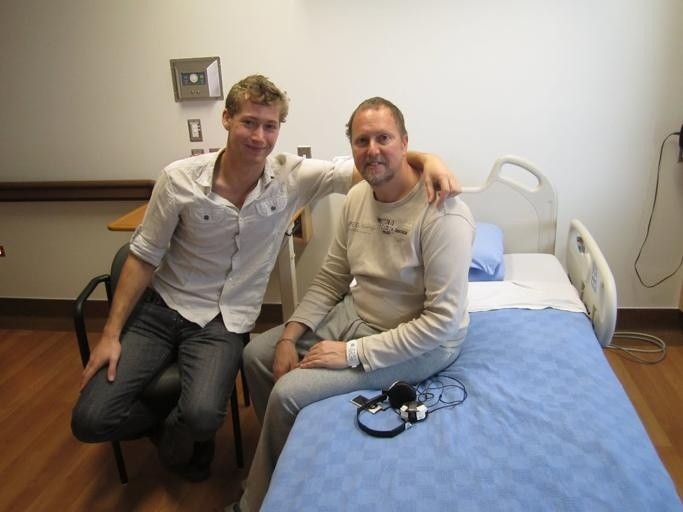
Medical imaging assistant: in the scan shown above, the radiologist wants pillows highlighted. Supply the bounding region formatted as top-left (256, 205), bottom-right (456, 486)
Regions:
top-left (466, 218), bottom-right (504, 282)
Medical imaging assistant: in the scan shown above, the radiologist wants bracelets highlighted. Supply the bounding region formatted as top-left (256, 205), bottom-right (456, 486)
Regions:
top-left (346, 340), bottom-right (360, 368)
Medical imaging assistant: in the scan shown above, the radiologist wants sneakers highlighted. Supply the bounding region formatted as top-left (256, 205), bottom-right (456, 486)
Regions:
top-left (150, 438), bottom-right (215, 482)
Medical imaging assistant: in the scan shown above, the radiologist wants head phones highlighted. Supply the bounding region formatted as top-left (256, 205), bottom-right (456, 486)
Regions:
top-left (357, 380), bottom-right (428, 438)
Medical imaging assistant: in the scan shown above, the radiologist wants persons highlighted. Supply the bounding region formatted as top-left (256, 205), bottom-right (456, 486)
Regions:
top-left (230, 97), bottom-right (475, 505)
top-left (73, 81), bottom-right (463, 483)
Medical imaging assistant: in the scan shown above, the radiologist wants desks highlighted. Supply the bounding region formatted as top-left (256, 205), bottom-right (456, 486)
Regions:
top-left (107, 203), bottom-right (313, 325)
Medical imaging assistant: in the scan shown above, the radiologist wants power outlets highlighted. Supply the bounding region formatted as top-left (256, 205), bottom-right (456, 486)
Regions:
top-left (191, 149), bottom-right (203, 156)
top-left (187, 119), bottom-right (203, 142)
top-left (297, 147), bottom-right (311, 159)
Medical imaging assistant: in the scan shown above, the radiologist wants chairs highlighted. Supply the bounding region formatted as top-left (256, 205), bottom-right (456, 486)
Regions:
top-left (70, 238), bottom-right (252, 484)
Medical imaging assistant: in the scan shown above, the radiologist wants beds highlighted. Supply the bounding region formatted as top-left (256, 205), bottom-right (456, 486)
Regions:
top-left (259, 153), bottom-right (683, 512)
